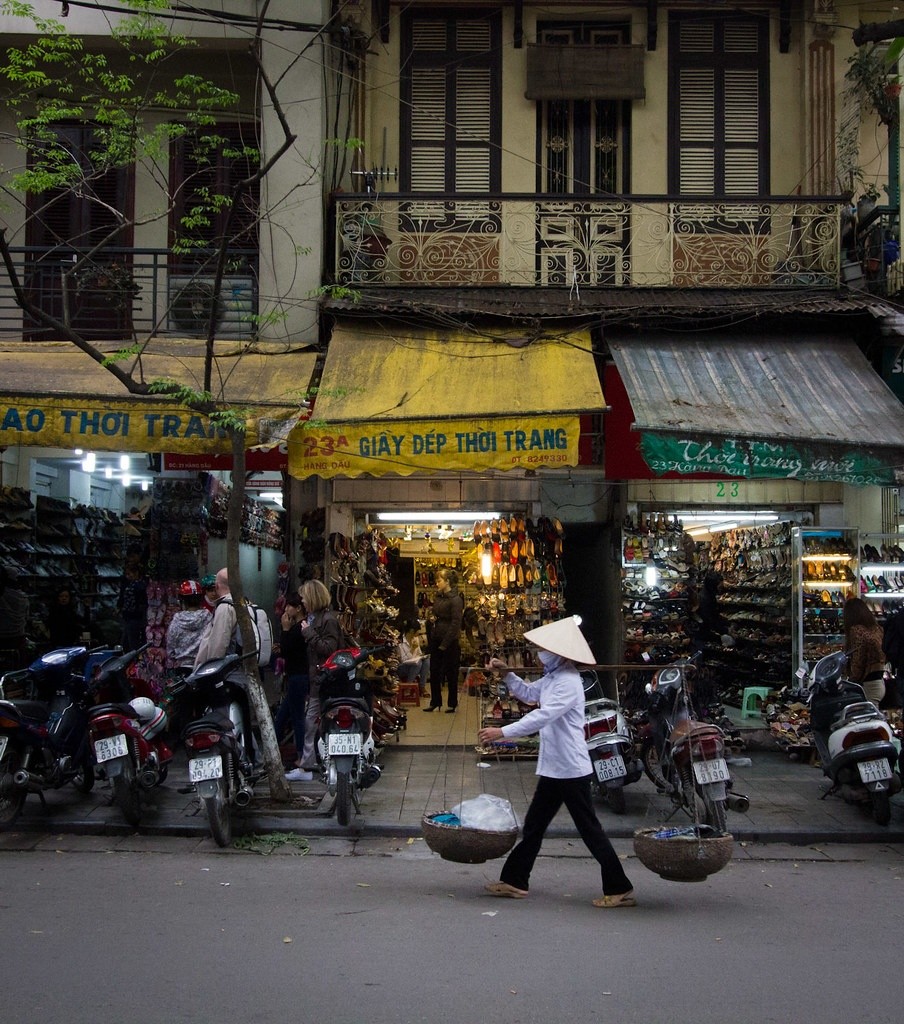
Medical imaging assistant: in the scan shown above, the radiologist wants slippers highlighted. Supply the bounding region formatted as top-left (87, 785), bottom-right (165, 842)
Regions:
top-left (592, 895), bottom-right (637, 907)
top-left (144, 581), bottom-right (203, 696)
top-left (483, 883), bottom-right (526, 899)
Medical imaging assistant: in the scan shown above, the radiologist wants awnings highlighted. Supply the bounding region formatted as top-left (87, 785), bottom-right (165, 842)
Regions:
top-left (288, 322), bottom-right (611, 480)
top-left (604, 336), bottom-right (904, 487)
top-left (0, 340), bottom-right (316, 454)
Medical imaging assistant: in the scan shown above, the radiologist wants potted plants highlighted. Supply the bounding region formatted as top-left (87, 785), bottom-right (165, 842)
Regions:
top-left (885, 75), bottom-right (904, 98)
top-left (839, 179), bottom-right (894, 289)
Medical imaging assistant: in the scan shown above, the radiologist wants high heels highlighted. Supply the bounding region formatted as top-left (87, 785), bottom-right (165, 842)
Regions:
top-left (419, 688), bottom-right (431, 698)
top-left (446, 704), bottom-right (455, 714)
top-left (423, 702), bottom-right (442, 712)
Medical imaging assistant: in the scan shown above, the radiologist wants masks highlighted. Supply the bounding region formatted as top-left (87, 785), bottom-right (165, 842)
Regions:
top-left (536, 649), bottom-right (558, 667)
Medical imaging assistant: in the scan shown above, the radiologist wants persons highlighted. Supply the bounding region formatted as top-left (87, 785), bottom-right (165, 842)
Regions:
top-left (422, 569), bottom-right (463, 712)
top-left (39, 587), bottom-right (91, 649)
top-left (478, 615), bottom-right (637, 907)
top-left (691, 572), bottom-right (723, 706)
top-left (0, 587), bottom-right (29, 668)
top-left (285, 579), bottom-right (345, 780)
top-left (114, 543), bottom-right (142, 653)
top-left (843, 599), bottom-right (886, 709)
top-left (164, 580), bottom-right (214, 750)
top-left (185, 568), bottom-right (247, 684)
top-left (397, 621), bottom-right (430, 698)
top-left (273, 592), bottom-right (310, 767)
top-left (882, 608), bottom-right (904, 708)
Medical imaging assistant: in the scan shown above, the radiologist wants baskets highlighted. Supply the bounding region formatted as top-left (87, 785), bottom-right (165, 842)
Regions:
top-left (634, 827), bottom-right (733, 883)
top-left (421, 811), bottom-right (518, 864)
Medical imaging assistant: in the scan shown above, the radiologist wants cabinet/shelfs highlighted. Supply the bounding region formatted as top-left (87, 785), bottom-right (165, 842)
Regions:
top-left (347, 586), bottom-right (398, 639)
top-left (0, 496), bottom-right (123, 627)
top-left (621, 519), bottom-right (904, 710)
top-left (479, 691), bottom-right (540, 762)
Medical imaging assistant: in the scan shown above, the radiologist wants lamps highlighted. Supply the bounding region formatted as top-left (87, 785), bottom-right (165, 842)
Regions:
top-left (256, 491), bottom-right (283, 498)
top-left (404, 527), bottom-right (454, 541)
top-left (687, 522), bottom-right (740, 536)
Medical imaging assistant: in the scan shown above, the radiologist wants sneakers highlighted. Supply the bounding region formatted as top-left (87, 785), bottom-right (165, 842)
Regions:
top-left (285, 768), bottom-right (313, 782)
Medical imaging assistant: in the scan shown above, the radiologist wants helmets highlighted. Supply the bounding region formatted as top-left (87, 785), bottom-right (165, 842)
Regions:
top-left (178, 582), bottom-right (201, 597)
top-left (129, 697), bottom-right (156, 721)
top-left (202, 574), bottom-right (219, 589)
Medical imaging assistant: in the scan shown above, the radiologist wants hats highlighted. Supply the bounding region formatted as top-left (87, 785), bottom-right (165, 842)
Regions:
top-left (522, 615), bottom-right (597, 666)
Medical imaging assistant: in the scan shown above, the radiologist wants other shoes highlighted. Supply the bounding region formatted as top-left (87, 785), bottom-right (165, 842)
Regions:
top-left (471, 514), bottom-right (569, 756)
top-left (0, 483), bottom-right (131, 650)
top-left (328, 529), bottom-right (408, 736)
top-left (624, 511), bottom-right (903, 752)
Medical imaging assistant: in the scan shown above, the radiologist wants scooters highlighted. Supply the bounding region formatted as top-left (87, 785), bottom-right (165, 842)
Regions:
top-left (576, 671), bottom-right (643, 815)
top-left (795, 639), bottom-right (904, 826)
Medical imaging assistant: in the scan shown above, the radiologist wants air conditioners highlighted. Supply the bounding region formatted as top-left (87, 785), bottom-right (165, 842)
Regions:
top-left (169, 275), bottom-right (252, 340)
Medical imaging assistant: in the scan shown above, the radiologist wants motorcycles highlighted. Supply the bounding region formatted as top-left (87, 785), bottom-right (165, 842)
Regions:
top-left (643, 650), bottom-right (749, 836)
top-left (307, 646), bottom-right (382, 823)
top-left (0, 641), bottom-right (177, 832)
top-left (167, 649), bottom-right (271, 846)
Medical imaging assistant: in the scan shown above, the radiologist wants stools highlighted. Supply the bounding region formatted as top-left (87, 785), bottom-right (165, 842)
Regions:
top-left (396, 675), bottom-right (421, 707)
top-left (741, 686), bottom-right (774, 721)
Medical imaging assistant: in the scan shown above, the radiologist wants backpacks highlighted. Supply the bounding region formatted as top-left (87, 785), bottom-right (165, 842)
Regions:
top-left (220, 596), bottom-right (273, 666)
